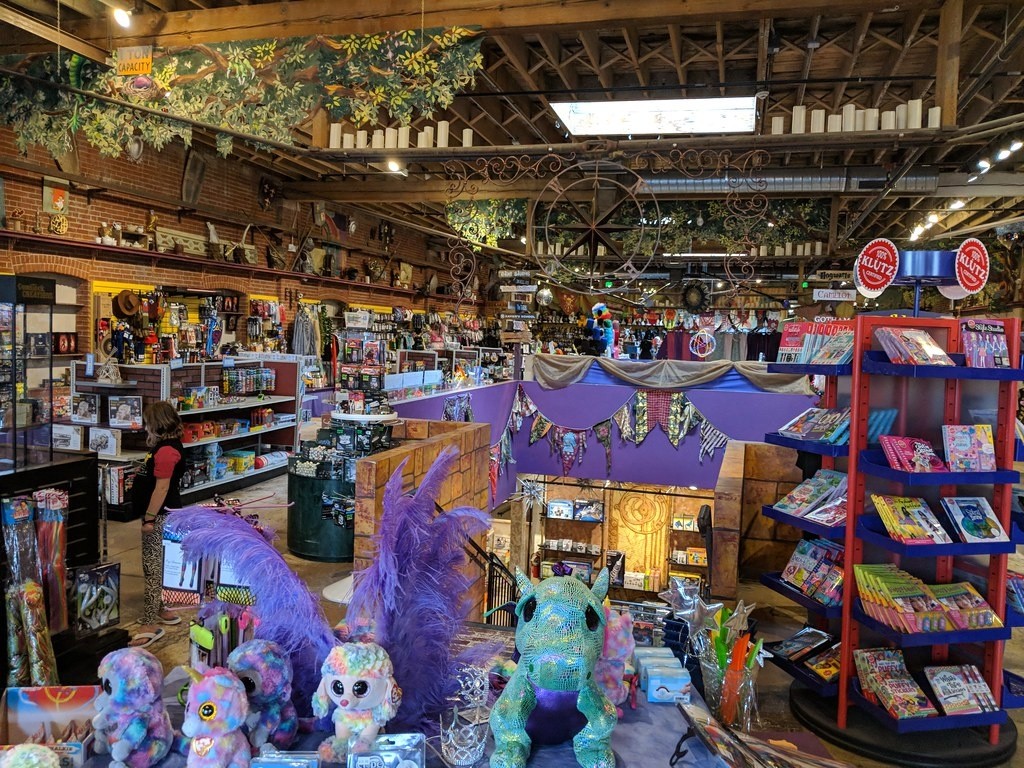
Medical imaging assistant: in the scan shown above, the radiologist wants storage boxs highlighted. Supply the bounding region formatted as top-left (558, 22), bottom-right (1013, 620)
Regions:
top-left (225, 449), bottom-right (255, 474)
top-left (53, 424), bottom-right (84, 452)
top-left (547, 500), bottom-right (573, 521)
top-left (110, 465), bottom-right (138, 505)
top-left (88, 427), bottom-right (120, 457)
top-left (633, 647), bottom-right (675, 673)
top-left (638, 657), bottom-right (683, 693)
top-left (576, 498), bottom-right (604, 522)
top-left (647, 668), bottom-right (690, 704)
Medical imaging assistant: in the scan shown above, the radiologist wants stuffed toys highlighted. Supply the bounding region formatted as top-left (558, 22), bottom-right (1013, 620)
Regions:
top-left (90, 445), bottom-right (619, 768)
top-left (580, 303), bottom-right (664, 362)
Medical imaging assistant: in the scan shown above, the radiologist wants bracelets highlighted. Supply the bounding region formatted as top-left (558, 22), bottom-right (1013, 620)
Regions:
top-left (145, 510), bottom-right (157, 518)
top-left (142, 518), bottom-right (154, 526)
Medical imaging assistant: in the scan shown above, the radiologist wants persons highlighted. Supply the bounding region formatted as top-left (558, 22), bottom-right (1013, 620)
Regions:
top-left (129, 402), bottom-right (186, 650)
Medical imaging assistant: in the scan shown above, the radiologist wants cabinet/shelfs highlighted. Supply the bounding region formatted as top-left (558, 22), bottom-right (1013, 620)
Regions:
top-left (53, 354), bottom-right (306, 522)
top-left (758, 308), bottom-right (1024, 768)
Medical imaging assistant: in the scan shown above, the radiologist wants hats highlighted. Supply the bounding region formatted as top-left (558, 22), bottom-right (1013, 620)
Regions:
top-left (118, 290), bottom-right (139, 315)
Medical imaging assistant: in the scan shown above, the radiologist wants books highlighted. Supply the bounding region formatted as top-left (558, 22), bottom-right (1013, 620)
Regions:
top-left (777, 319), bottom-right (1011, 369)
top-left (767, 408), bottom-right (1010, 721)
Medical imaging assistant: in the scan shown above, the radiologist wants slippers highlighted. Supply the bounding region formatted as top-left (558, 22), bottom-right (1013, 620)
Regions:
top-left (128, 628), bottom-right (165, 647)
top-left (137, 614), bottom-right (182, 624)
